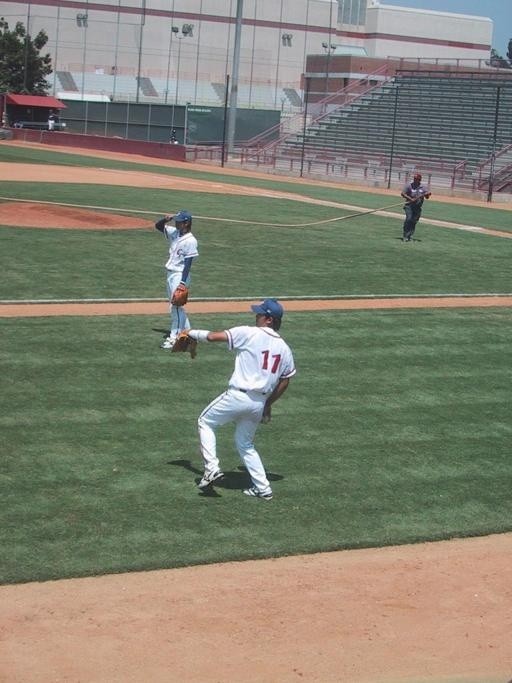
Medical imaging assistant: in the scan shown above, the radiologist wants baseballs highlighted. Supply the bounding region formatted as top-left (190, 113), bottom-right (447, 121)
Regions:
top-left (262, 415), bottom-right (269, 424)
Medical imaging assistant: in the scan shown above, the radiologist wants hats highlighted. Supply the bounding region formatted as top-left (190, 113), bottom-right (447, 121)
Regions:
top-left (414, 174), bottom-right (421, 180)
top-left (172, 209), bottom-right (193, 222)
top-left (250, 298), bottom-right (283, 320)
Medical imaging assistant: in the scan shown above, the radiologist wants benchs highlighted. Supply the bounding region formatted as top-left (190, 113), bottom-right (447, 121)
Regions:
top-left (278, 70), bottom-right (512, 181)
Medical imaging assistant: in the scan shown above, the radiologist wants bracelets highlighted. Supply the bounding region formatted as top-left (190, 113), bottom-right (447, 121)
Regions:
top-left (188, 328), bottom-right (213, 343)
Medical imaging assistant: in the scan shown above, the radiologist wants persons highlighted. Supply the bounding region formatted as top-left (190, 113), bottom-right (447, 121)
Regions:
top-left (399, 172), bottom-right (433, 240)
top-left (48, 110), bottom-right (58, 129)
top-left (171, 296), bottom-right (298, 501)
top-left (167, 127), bottom-right (177, 141)
top-left (154, 209), bottom-right (197, 347)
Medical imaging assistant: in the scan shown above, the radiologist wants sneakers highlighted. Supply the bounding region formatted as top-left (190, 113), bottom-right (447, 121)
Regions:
top-left (242, 488), bottom-right (273, 501)
top-left (197, 467), bottom-right (224, 488)
top-left (160, 336), bottom-right (178, 349)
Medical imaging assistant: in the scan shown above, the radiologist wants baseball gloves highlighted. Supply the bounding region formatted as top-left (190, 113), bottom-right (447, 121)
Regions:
top-left (170, 285), bottom-right (188, 306)
top-left (171, 330), bottom-right (198, 360)
